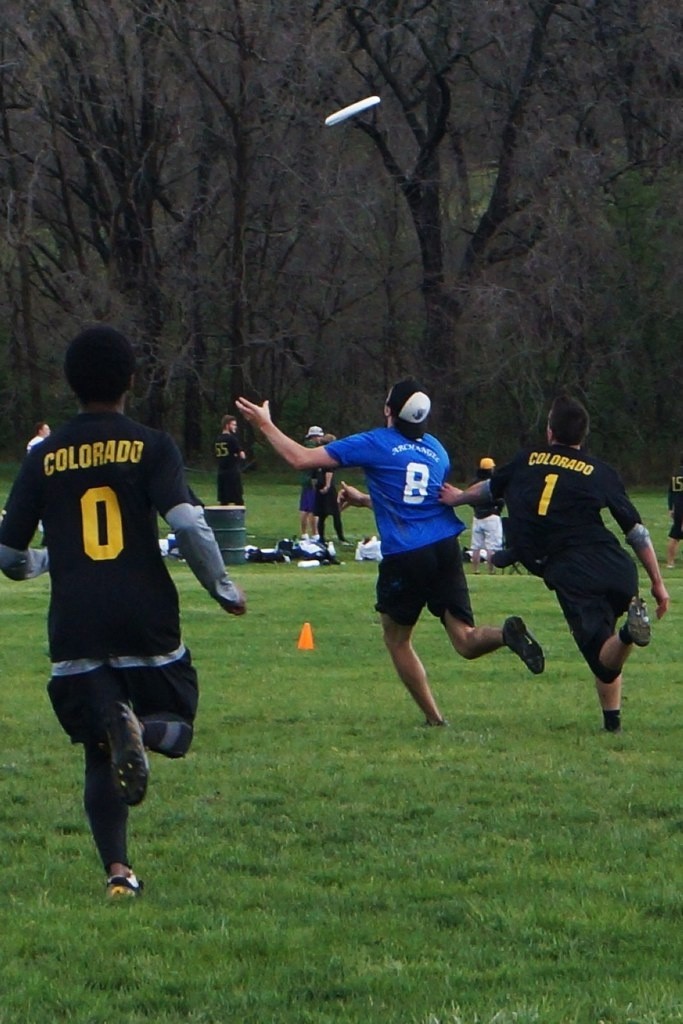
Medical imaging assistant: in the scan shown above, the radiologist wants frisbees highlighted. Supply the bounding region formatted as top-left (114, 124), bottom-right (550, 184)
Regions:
top-left (325, 94), bottom-right (382, 128)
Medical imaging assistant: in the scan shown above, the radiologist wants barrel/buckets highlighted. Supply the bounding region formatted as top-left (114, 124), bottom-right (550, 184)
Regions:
top-left (204, 505), bottom-right (246, 565)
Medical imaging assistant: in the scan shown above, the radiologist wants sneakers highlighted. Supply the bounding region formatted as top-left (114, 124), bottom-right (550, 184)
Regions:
top-left (100, 699), bottom-right (149, 807)
top-left (420, 719), bottom-right (449, 728)
top-left (596, 728), bottom-right (623, 733)
top-left (626, 596), bottom-right (651, 647)
top-left (106, 870), bottom-right (144, 902)
top-left (501, 617), bottom-right (545, 674)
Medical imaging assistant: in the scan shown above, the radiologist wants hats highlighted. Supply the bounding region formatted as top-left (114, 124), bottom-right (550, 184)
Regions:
top-left (320, 433), bottom-right (336, 443)
top-left (480, 458), bottom-right (495, 470)
top-left (386, 380), bottom-right (432, 441)
top-left (305, 426), bottom-right (324, 438)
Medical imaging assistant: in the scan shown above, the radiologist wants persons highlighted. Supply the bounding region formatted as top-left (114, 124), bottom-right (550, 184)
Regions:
top-left (214, 415), bottom-right (245, 506)
top-left (299, 426), bottom-right (324, 540)
top-left (437, 395), bottom-right (671, 734)
top-left (313, 434), bottom-right (355, 545)
top-left (235, 381), bottom-right (545, 727)
top-left (466, 458), bottom-right (502, 575)
top-left (27, 422), bottom-right (51, 531)
top-left (667, 454), bottom-right (683, 568)
top-left (0, 326), bottom-right (246, 899)
